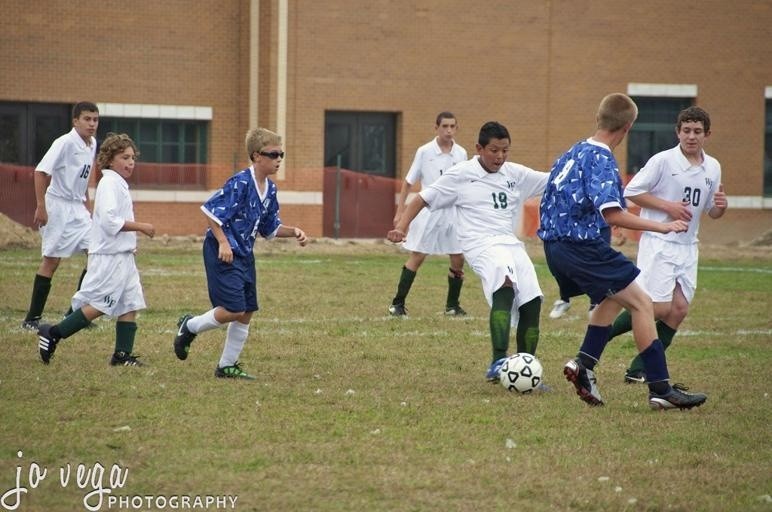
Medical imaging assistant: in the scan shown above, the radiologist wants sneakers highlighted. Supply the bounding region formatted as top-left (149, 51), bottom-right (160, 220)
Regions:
top-left (565, 361), bottom-right (602, 405)
top-left (215, 362), bottom-right (257, 380)
top-left (627, 369), bottom-right (707, 410)
top-left (485, 358), bottom-right (505, 382)
top-left (112, 352), bottom-right (147, 368)
top-left (388, 303), bottom-right (408, 320)
top-left (444, 306), bottom-right (467, 316)
top-left (175, 312), bottom-right (197, 360)
top-left (21, 316), bottom-right (95, 362)
top-left (549, 300), bottom-right (573, 319)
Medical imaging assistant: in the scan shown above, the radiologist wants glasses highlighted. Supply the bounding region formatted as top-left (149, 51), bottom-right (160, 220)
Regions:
top-left (258, 152), bottom-right (284, 159)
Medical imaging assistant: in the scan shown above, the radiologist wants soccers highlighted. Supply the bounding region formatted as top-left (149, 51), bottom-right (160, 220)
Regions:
top-left (499, 353), bottom-right (545, 394)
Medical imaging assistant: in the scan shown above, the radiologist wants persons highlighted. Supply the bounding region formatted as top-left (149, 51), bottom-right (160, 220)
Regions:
top-left (537, 91), bottom-right (706, 411)
top-left (607, 107), bottom-right (729, 385)
top-left (173, 127), bottom-right (308, 380)
top-left (388, 113), bottom-right (469, 317)
top-left (546, 291), bottom-right (599, 319)
top-left (36, 133), bottom-right (157, 368)
top-left (385, 122), bottom-right (551, 391)
top-left (22, 102), bottom-right (103, 332)
